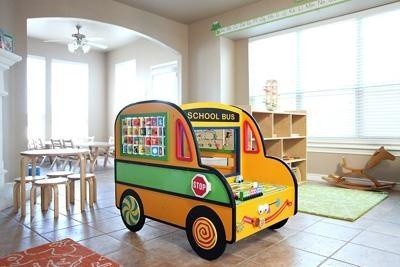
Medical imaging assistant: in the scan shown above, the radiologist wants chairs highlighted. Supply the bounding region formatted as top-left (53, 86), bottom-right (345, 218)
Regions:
top-left (27, 137), bottom-right (115, 172)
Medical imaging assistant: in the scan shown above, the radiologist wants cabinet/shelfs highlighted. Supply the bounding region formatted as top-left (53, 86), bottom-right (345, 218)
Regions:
top-left (252, 110), bottom-right (307, 187)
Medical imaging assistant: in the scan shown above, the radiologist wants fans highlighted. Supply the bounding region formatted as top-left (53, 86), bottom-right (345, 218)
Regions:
top-left (42, 24), bottom-right (108, 50)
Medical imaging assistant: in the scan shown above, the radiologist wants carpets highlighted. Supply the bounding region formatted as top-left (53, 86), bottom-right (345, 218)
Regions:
top-left (297, 180), bottom-right (389, 222)
top-left (1, 236), bottom-right (119, 266)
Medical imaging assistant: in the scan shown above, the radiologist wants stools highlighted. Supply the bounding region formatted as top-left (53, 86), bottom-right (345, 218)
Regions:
top-left (12, 170), bottom-right (97, 217)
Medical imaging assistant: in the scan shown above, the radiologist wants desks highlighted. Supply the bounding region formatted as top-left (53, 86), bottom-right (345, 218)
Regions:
top-left (19, 147), bottom-right (94, 217)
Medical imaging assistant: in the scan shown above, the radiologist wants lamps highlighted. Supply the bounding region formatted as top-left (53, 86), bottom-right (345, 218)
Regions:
top-left (68, 38), bottom-right (91, 54)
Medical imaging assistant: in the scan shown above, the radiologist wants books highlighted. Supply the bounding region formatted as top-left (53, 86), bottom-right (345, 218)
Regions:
top-left (284, 155), bottom-right (300, 161)
